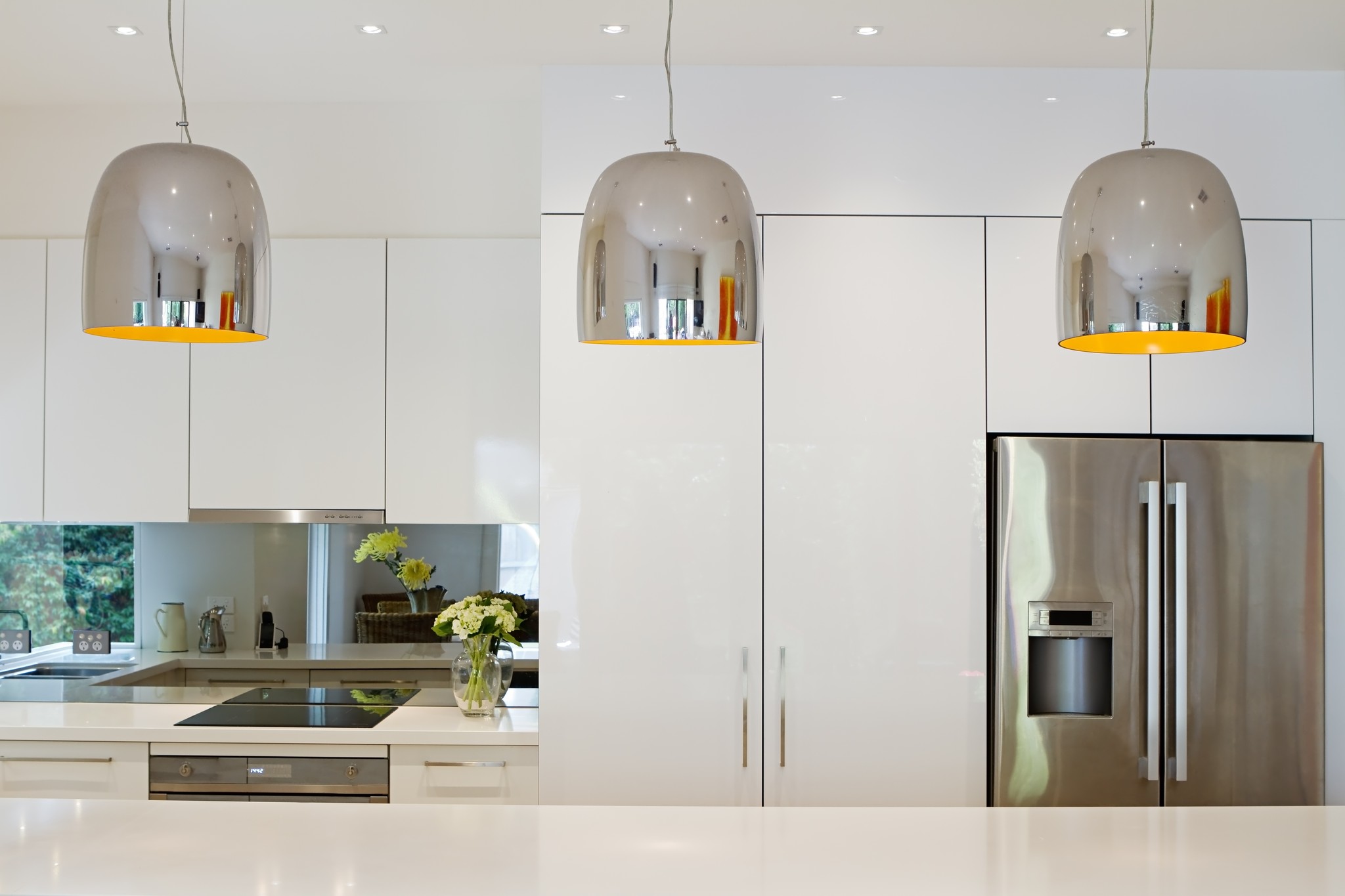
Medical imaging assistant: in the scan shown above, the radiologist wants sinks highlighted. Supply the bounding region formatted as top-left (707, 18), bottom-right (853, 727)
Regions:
top-left (0, 662), bottom-right (137, 680)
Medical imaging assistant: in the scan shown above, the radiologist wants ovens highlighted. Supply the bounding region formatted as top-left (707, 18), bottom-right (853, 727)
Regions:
top-left (149, 757), bottom-right (390, 804)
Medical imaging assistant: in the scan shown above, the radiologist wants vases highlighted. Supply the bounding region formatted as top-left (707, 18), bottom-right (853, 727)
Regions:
top-left (406, 585), bottom-right (448, 613)
top-left (452, 636), bottom-right (502, 718)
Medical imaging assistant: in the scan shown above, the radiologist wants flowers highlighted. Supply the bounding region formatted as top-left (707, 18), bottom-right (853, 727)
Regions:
top-left (353, 527), bottom-right (436, 590)
top-left (432, 595), bottom-right (527, 648)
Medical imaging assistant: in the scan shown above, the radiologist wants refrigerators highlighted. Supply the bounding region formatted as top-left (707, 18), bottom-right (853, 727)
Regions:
top-left (989, 434), bottom-right (1327, 806)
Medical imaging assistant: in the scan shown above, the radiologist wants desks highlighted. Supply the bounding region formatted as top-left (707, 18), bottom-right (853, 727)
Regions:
top-left (0, 798), bottom-right (1345, 896)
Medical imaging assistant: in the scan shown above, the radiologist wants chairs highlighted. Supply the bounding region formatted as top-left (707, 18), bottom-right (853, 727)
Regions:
top-left (356, 593), bottom-right (539, 643)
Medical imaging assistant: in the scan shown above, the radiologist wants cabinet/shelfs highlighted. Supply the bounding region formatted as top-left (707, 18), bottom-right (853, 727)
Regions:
top-left (0, 642), bottom-right (538, 707)
top-left (0, 701), bottom-right (538, 805)
top-left (538, 65), bottom-right (1345, 807)
top-left (0, 239), bottom-right (539, 523)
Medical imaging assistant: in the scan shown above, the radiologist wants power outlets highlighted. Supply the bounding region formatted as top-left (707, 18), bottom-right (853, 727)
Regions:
top-left (73, 630), bottom-right (111, 654)
top-left (208, 596), bottom-right (235, 632)
top-left (0, 629), bottom-right (31, 653)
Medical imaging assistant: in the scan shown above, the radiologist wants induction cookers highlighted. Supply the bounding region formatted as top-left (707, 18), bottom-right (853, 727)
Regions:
top-left (222, 687), bottom-right (422, 706)
top-left (174, 705), bottom-right (399, 728)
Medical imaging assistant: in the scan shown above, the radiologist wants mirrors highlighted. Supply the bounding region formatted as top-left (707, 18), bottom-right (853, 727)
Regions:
top-left (0, 522), bottom-right (539, 706)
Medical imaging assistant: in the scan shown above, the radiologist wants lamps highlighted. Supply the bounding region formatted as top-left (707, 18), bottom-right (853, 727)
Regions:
top-left (575, 0), bottom-right (764, 345)
top-left (1054, 0), bottom-right (1248, 354)
top-left (81, 0), bottom-right (270, 343)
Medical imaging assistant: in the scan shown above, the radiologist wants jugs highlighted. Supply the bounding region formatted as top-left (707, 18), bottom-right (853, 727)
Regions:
top-left (153, 602), bottom-right (189, 652)
top-left (197, 606), bottom-right (228, 653)
